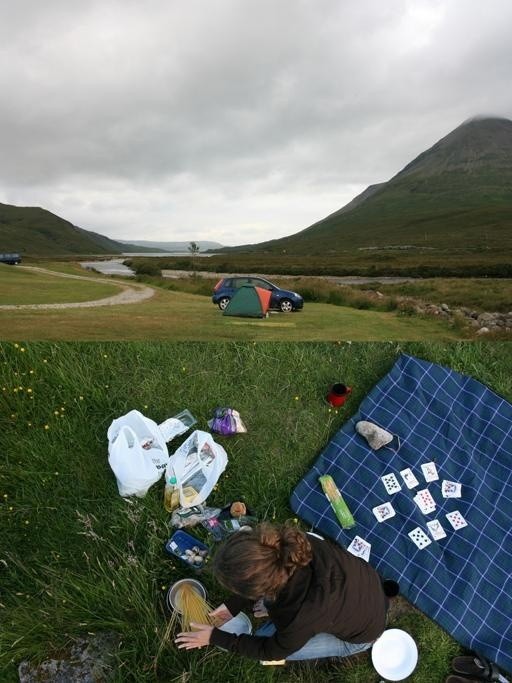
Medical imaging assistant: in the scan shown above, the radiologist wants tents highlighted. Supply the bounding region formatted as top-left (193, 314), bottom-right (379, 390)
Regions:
top-left (223, 282), bottom-right (272, 318)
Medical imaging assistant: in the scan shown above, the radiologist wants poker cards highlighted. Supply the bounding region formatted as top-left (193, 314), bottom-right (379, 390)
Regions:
top-left (348, 536), bottom-right (371, 562)
top-left (371, 462), bottom-right (469, 550)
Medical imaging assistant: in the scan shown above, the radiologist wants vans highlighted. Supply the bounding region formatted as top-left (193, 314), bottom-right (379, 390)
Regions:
top-left (0, 252), bottom-right (22, 265)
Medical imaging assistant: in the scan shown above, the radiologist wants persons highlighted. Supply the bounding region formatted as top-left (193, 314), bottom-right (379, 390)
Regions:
top-left (174, 520), bottom-right (390, 661)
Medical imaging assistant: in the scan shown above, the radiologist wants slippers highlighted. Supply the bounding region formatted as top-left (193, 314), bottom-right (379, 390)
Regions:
top-left (445, 655), bottom-right (499, 683)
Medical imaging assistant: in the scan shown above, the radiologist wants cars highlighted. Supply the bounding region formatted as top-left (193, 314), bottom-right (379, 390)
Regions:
top-left (212, 276), bottom-right (304, 312)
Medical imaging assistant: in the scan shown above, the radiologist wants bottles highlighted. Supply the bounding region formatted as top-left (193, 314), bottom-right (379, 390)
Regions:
top-left (164, 476), bottom-right (180, 512)
top-left (328, 383), bottom-right (351, 407)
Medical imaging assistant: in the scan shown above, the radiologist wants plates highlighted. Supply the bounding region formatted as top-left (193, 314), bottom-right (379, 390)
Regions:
top-left (371, 628), bottom-right (419, 681)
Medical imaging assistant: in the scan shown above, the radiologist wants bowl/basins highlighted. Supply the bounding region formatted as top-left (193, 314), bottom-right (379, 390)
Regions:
top-left (169, 578), bottom-right (206, 615)
